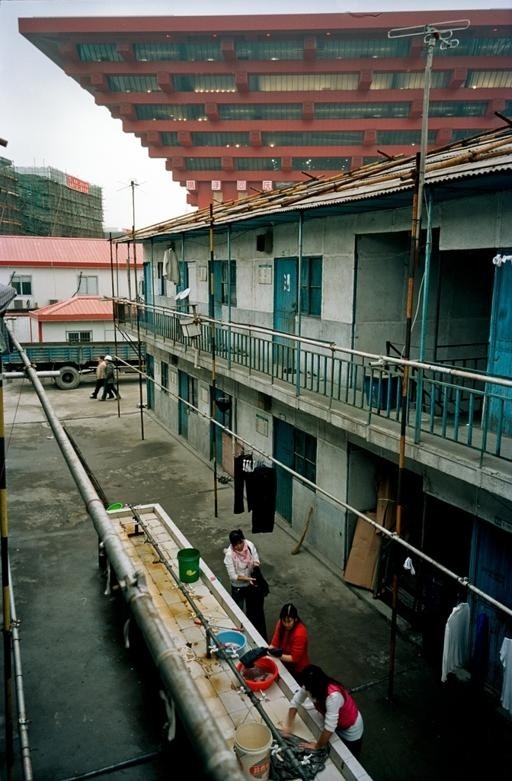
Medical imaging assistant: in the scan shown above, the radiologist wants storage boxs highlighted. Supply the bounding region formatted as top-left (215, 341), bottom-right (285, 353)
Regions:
top-left (365, 373), bottom-right (403, 409)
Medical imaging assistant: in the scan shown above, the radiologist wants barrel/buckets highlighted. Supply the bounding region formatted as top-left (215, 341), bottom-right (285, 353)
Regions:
top-left (234, 723), bottom-right (272, 781)
top-left (178, 548), bottom-right (200, 582)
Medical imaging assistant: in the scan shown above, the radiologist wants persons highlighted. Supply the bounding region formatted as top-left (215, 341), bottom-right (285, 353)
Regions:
top-left (89, 356), bottom-right (115, 400)
top-left (224, 529), bottom-right (261, 622)
top-left (266, 604), bottom-right (311, 688)
top-left (280, 665), bottom-right (364, 762)
top-left (98, 355), bottom-right (121, 402)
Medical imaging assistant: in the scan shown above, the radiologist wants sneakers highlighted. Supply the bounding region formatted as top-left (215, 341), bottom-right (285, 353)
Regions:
top-left (90, 394), bottom-right (121, 401)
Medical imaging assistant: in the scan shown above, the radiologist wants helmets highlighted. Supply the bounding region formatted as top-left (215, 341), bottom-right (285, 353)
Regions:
top-left (104, 355), bottom-right (112, 361)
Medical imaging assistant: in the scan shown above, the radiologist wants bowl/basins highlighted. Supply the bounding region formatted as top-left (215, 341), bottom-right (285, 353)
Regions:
top-left (236, 655), bottom-right (278, 692)
top-left (107, 503), bottom-right (124, 512)
top-left (211, 630), bottom-right (247, 659)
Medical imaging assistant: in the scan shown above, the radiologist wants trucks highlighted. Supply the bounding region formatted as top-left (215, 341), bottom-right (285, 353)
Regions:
top-left (1, 281), bottom-right (146, 389)
